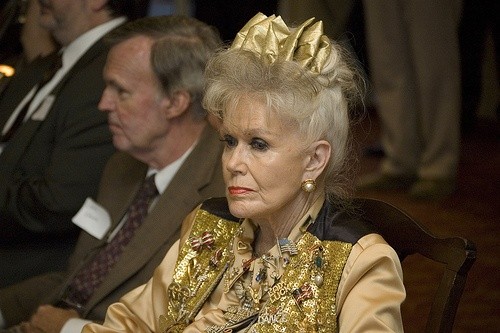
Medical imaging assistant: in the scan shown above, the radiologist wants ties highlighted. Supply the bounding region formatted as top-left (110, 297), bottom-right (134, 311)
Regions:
top-left (0, 50), bottom-right (63, 145)
top-left (55, 174), bottom-right (156, 317)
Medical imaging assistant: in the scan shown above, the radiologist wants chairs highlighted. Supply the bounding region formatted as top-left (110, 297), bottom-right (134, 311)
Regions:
top-left (339, 196), bottom-right (479, 333)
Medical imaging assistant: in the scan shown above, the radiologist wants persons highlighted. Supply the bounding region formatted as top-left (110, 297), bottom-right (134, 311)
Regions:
top-left (0, 12), bottom-right (226, 333)
top-left (0, 0), bottom-right (500, 287)
top-left (59, 11), bottom-right (407, 333)
